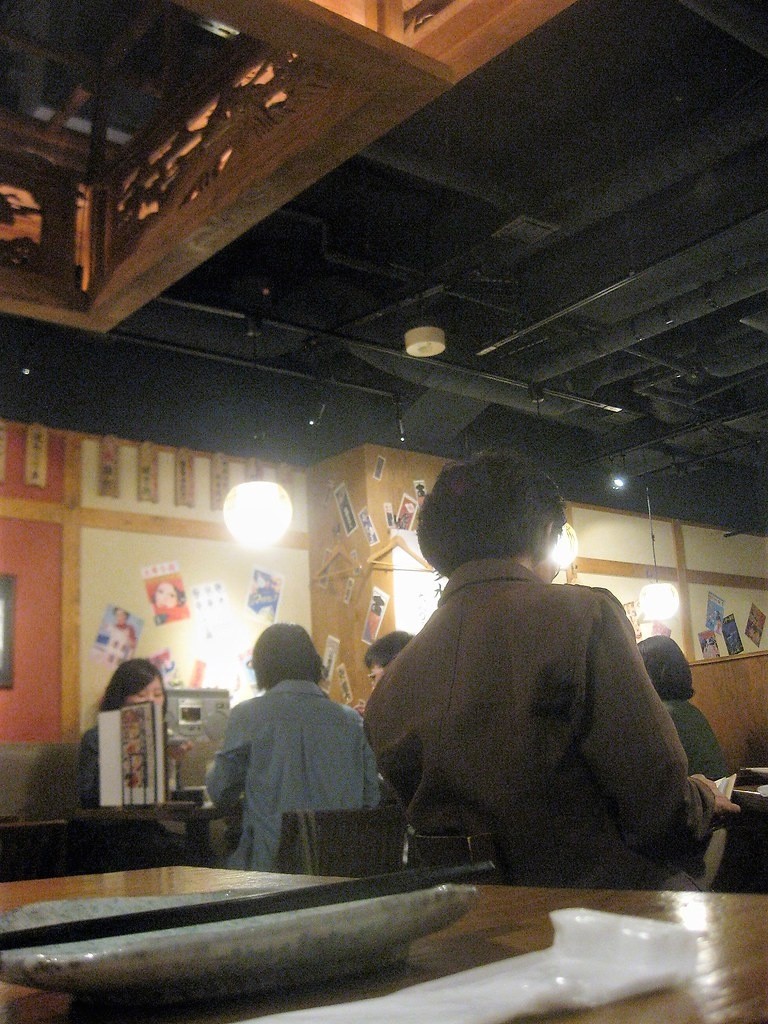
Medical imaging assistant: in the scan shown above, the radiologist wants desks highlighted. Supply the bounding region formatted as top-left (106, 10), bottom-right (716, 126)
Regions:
top-left (0, 867), bottom-right (768, 1024)
top-left (0, 816), bottom-right (67, 884)
top-left (75, 787), bottom-right (243, 865)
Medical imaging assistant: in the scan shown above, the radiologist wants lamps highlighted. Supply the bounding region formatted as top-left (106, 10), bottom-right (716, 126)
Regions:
top-left (223, 308), bottom-right (293, 554)
top-left (521, 381), bottom-right (579, 573)
top-left (638, 487), bottom-right (682, 624)
top-left (402, 219), bottom-right (448, 359)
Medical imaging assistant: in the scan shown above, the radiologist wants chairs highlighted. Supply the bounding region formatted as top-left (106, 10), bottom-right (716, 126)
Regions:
top-left (700, 771), bottom-right (765, 829)
top-left (404, 827), bottom-right (515, 888)
top-left (273, 806), bottom-right (401, 878)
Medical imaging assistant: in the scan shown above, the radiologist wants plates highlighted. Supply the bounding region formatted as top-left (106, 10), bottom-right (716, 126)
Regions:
top-left (1, 882), bottom-right (480, 1005)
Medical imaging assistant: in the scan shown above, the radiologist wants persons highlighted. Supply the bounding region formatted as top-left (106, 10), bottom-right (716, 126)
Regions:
top-left (352, 631), bottom-right (416, 720)
top-left (634, 636), bottom-right (730, 785)
top-left (74, 656), bottom-right (193, 808)
top-left (357, 450), bottom-right (739, 896)
top-left (207, 622), bottom-right (380, 876)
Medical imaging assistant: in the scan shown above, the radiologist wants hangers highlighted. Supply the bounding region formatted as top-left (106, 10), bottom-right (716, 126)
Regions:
top-left (311, 518), bottom-right (437, 582)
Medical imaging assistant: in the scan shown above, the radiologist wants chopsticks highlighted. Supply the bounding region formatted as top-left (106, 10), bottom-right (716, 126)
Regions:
top-left (0, 856), bottom-right (497, 951)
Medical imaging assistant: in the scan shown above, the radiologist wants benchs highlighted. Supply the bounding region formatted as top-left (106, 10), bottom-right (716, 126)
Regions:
top-left (0, 737), bottom-right (239, 868)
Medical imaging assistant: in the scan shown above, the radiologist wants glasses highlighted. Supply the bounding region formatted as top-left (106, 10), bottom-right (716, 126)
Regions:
top-left (367, 667), bottom-right (383, 679)
